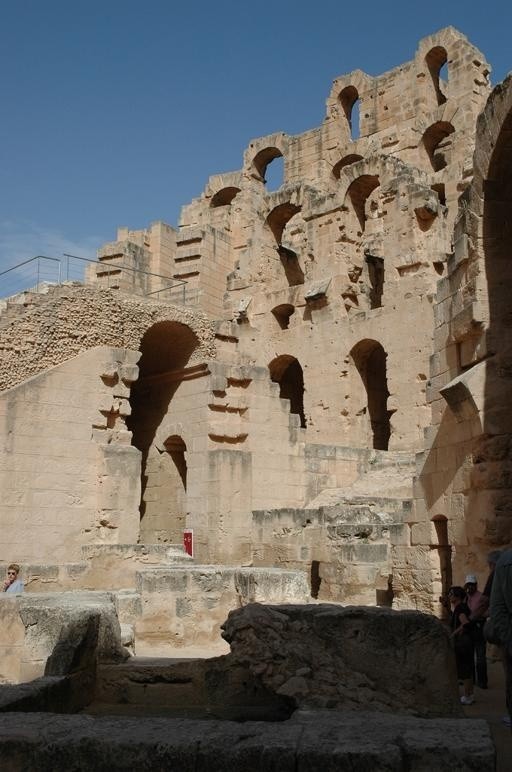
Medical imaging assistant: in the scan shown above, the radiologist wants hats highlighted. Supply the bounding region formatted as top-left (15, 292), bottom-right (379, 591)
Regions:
top-left (465, 575), bottom-right (476, 584)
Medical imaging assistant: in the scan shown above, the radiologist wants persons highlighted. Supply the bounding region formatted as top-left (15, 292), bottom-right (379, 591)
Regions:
top-left (447, 548), bottom-right (512, 727)
top-left (1, 564), bottom-right (24, 593)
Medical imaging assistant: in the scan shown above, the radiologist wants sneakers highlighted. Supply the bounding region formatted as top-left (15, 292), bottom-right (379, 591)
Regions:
top-left (476, 681), bottom-right (488, 689)
top-left (501, 715), bottom-right (511, 727)
top-left (461, 696), bottom-right (475, 704)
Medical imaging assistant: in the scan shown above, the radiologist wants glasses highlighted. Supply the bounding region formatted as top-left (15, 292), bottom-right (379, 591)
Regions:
top-left (8, 572), bottom-right (15, 575)
top-left (448, 592), bottom-right (453, 596)
